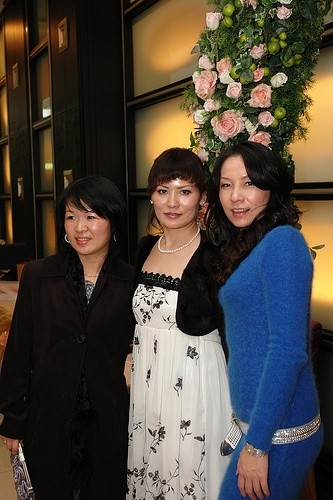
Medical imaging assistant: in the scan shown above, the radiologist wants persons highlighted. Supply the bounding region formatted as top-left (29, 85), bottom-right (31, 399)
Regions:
top-left (210, 142), bottom-right (324, 500)
top-left (0, 175), bottom-right (141, 500)
top-left (126, 148), bottom-right (231, 500)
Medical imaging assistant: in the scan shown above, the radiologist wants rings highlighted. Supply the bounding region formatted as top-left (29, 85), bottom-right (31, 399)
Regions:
top-left (3, 440), bottom-right (6, 442)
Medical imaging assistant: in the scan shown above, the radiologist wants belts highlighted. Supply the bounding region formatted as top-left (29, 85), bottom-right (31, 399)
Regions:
top-left (220, 412), bottom-right (322, 456)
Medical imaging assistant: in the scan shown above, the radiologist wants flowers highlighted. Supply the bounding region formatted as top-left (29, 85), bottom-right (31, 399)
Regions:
top-left (177, 0), bottom-right (333, 231)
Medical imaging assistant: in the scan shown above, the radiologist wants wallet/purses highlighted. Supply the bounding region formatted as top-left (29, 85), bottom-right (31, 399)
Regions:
top-left (9, 442), bottom-right (34, 500)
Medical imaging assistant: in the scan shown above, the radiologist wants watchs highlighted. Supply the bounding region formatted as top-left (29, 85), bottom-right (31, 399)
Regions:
top-left (245, 443), bottom-right (267, 457)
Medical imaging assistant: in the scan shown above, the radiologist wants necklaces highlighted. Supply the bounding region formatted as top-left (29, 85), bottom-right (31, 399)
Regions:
top-left (84, 273), bottom-right (99, 277)
top-left (157, 224), bottom-right (200, 253)
